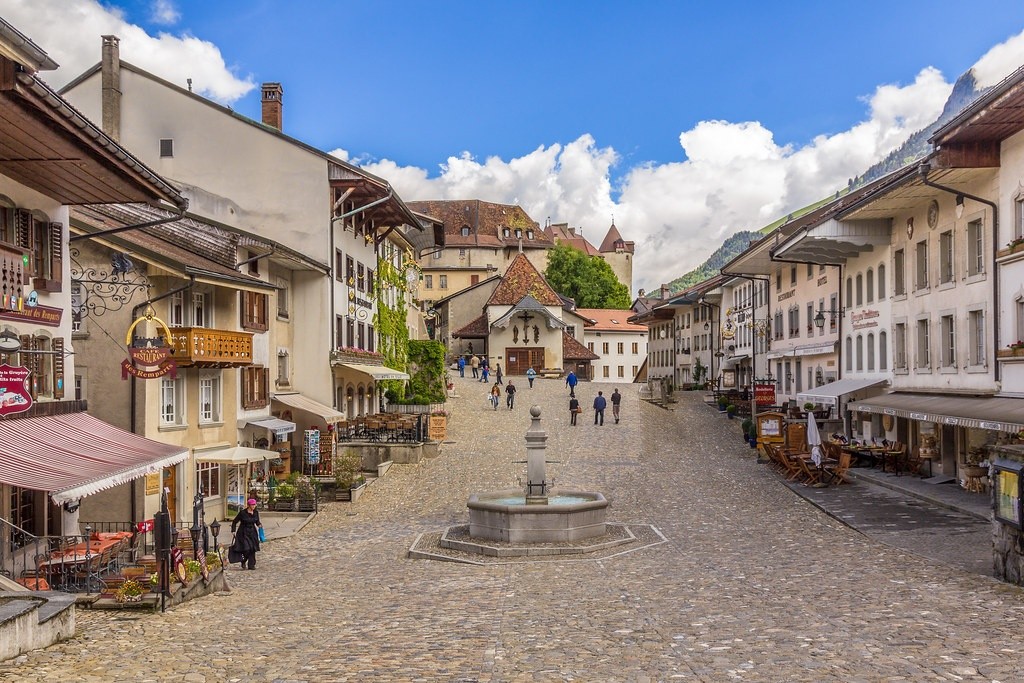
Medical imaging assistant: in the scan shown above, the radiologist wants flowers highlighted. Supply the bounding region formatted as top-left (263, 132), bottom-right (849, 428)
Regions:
top-left (113, 578), bottom-right (145, 598)
top-left (432, 409), bottom-right (448, 415)
top-left (150, 551), bottom-right (222, 583)
top-left (1007, 339), bottom-right (1024, 351)
top-left (1006, 236), bottom-right (1024, 254)
top-left (339, 345), bottom-right (382, 357)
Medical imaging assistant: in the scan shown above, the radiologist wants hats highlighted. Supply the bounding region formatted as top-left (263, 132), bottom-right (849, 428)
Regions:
top-left (248, 499), bottom-right (256, 505)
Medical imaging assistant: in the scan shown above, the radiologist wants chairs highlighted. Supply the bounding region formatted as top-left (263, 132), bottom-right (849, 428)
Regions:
top-left (338, 411), bottom-right (429, 445)
top-left (781, 402), bottom-right (831, 427)
top-left (762, 441), bottom-right (857, 487)
top-left (873, 439), bottom-right (927, 477)
top-left (0, 530), bottom-right (143, 592)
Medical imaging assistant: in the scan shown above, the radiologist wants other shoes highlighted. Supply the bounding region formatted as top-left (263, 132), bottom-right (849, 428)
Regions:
top-left (250, 566), bottom-right (256, 570)
top-left (616, 419), bottom-right (619, 424)
top-left (242, 561), bottom-right (246, 568)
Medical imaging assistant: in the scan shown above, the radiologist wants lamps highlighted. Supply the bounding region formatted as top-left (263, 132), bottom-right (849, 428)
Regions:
top-left (189, 521), bottom-right (202, 541)
top-left (344, 393), bottom-right (352, 401)
top-left (786, 371), bottom-right (794, 383)
top-left (704, 321), bottom-right (720, 330)
top-left (169, 528), bottom-right (179, 546)
top-left (355, 391), bottom-right (372, 400)
top-left (816, 370), bottom-right (825, 386)
top-left (768, 372), bottom-right (777, 382)
top-left (210, 517), bottom-right (222, 537)
top-left (812, 310), bottom-right (846, 328)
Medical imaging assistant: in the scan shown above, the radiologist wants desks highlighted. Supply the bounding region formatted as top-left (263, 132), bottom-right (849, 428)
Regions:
top-left (796, 411), bottom-right (827, 417)
top-left (91, 531), bottom-right (133, 540)
top-left (15, 577), bottom-right (50, 591)
top-left (67, 539), bottom-right (120, 553)
top-left (772, 440), bottom-right (904, 483)
top-left (39, 554), bottom-right (97, 590)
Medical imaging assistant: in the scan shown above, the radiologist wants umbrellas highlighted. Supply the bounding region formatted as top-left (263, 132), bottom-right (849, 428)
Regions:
top-left (806, 412), bottom-right (828, 487)
top-left (197, 446), bottom-right (280, 510)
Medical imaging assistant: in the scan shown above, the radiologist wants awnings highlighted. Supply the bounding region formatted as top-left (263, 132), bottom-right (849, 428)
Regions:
top-left (274, 393), bottom-right (345, 424)
top-left (247, 418), bottom-right (296, 434)
top-left (727, 355), bottom-right (748, 364)
top-left (767, 339), bottom-right (838, 359)
top-left (797, 376), bottom-right (886, 404)
top-left (846, 394), bottom-right (1024, 433)
top-left (0, 414), bottom-right (189, 535)
top-left (338, 364), bottom-right (410, 380)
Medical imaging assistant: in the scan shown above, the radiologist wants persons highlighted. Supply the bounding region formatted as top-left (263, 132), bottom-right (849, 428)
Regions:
top-left (611, 389), bottom-right (621, 423)
top-left (566, 371), bottom-right (578, 397)
top-left (231, 499), bottom-right (263, 569)
top-left (496, 363), bottom-right (503, 385)
top-left (569, 396), bottom-right (579, 426)
top-left (492, 383), bottom-right (501, 411)
top-left (478, 356), bottom-right (489, 382)
top-left (505, 380), bottom-right (516, 409)
top-left (594, 391), bottom-right (606, 425)
top-left (470, 354), bottom-right (480, 378)
top-left (459, 355), bottom-right (465, 377)
top-left (526, 366), bottom-right (536, 390)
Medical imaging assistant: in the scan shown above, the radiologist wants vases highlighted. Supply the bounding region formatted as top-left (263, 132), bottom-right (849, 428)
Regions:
top-left (998, 249), bottom-right (1011, 257)
top-left (209, 564), bottom-right (216, 574)
top-left (1015, 348), bottom-right (1024, 356)
top-left (1013, 243), bottom-right (1024, 253)
top-left (432, 413), bottom-right (438, 417)
top-left (997, 350), bottom-right (1014, 356)
top-left (116, 594), bottom-right (143, 603)
top-left (152, 585), bottom-right (158, 592)
top-left (190, 571), bottom-right (197, 581)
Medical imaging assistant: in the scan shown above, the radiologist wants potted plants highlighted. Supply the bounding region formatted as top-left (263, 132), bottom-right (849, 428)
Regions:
top-left (332, 447), bottom-right (366, 501)
top-left (274, 473), bottom-right (321, 512)
top-left (726, 405), bottom-right (736, 419)
top-left (718, 395), bottom-right (727, 412)
top-left (703, 383), bottom-right (713, 391)
top-left (742, 419), bottom-right (757, 448)
top-left (271, 447), bottom-right (292, 472)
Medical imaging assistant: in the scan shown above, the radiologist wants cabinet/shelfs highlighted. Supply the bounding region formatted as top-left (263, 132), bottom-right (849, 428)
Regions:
top-left (301, 430), bottom-right (336, 474)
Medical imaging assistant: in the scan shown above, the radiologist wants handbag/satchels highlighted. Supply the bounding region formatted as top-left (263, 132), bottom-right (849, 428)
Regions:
top-left (259, 527), bottom-right (265, 542)
top-left (577, 406), bottom-right (582, 412)
top-left (489, 393), bottom-right (493, 400)
top-left (228, 537), bottom-right (243, 563)
